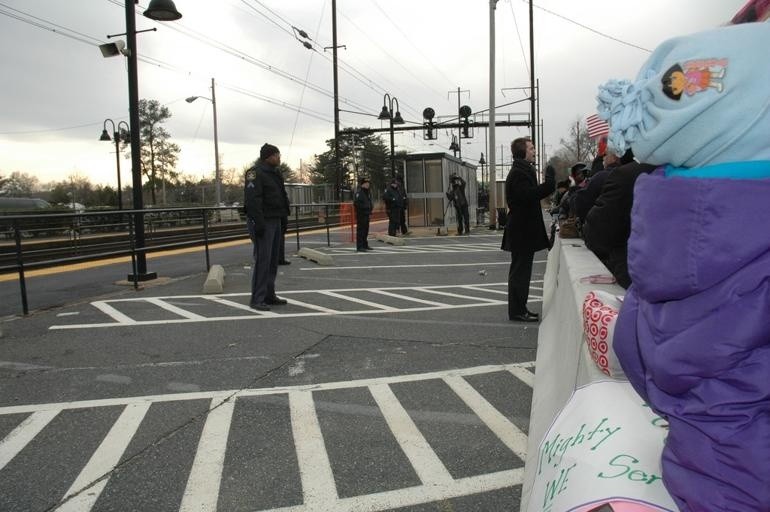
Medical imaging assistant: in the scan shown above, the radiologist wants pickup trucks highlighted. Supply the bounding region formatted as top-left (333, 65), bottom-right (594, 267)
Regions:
top-left (64, 203), bottom-right (85, 209)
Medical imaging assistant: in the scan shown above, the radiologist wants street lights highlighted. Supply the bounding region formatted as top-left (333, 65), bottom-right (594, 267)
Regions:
top-left (123, 0), bottom-right (184, 282)
top-left (180, 191), bottom-right (184, 200)
top-left (99, 119), bottom-right (131, 222)
top-left (186, 79), bottom-right (220, 207)
top-left (377, 94), bottom-right (405, 185)
top-left (448, 134), bottom-right (460, 158)
top-left (478, 151), bottom-right (487, 191)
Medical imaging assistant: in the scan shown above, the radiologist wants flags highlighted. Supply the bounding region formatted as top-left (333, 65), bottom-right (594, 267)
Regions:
top-left (586, 112), bottom-right (611, 138)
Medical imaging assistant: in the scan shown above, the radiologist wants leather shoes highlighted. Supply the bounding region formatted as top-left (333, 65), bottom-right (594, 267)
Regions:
top-left (510, 309), bottom-right (538, 323)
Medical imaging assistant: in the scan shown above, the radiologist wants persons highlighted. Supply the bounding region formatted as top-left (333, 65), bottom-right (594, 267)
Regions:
top-left (544, 138), bottom-right (661, 290)
top-left (353, 178), bottom-right (374, 251)
top-left (500, 135), bottom-right (558, 322)
top-left (383, 180), bottom-right (401, 237)
top-left (242, 142), bottom-right (293, 313)
top-left (595, 15), bottom-right (770, 512)
top-left (394, 171), bottom-right (412, 237)
top-left (445, 174), bottom-right (471, 236)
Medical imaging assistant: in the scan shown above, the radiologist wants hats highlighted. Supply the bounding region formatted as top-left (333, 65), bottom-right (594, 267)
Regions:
top-left (596, 22), bottom-right (769, 167)
top-left (260, 142), bottom-right (279, 158)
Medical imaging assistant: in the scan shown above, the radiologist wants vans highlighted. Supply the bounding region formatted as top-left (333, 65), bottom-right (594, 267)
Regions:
top-left (0, 197), bottom-right (51, 210)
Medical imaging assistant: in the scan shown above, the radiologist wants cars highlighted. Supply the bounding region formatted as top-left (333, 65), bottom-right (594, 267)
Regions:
top-left (232, 202), bottom-right (240, 207)
top-left (215, 203), bottom-right (226, 207)
top-left (92, 205), bottom-right (112, 210)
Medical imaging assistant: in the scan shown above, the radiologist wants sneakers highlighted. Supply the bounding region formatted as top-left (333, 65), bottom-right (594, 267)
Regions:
top-left (266, 297), bottom-right (287, 306)
top-left (252, 302), bottom-right (271, 311)
